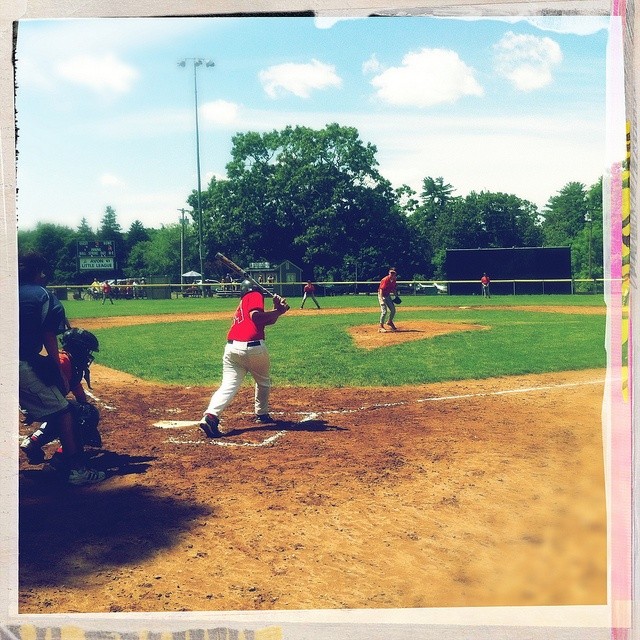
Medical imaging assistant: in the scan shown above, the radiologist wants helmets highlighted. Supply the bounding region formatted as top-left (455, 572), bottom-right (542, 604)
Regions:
top-left (63, 327), bottom-right (99, 369)
top-left (240, 279), bottom-right (263, 298)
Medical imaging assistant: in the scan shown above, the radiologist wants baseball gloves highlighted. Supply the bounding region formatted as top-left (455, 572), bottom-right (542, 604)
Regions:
top-left (392, 295), bottom-right (401, 304)
top-left (78, 403), bottom-right (101, 446)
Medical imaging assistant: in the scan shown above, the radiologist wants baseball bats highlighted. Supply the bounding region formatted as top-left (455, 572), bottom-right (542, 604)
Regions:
top-left (214, 251), bottom-right (291, 311)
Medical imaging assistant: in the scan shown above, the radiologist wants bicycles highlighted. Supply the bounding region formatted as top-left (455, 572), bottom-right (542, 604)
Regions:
top-left (83, 289), bottom-right (96, 301)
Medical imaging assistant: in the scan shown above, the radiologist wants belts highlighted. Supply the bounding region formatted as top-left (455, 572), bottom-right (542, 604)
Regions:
top-left (226, 338), bottom-right (264, 347)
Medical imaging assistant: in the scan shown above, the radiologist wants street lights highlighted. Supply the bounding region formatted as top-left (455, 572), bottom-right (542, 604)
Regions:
top-left (177, 57), bottom-right (214, 290)
top-left (178, 208), bottom-right (190, 290)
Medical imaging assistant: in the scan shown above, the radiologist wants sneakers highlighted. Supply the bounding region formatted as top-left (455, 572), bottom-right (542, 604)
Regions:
top-left (378, 327), bottom-right (387, 333)
top-left (386, 322), bottom-right (397, 330)
top-left (199, 416), bottom-right (223, 437)
top-left (255, 415), bottom-right (273, 424)
top-left (20, 436), bottom-right (44, 462)
top-left (69, 467), bottom-right (105, 487)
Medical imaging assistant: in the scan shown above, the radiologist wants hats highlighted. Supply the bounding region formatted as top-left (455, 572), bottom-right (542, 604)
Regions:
top-left (19, 252), bottom-right (55, 270)
top-left (389, 267), bottom-right (396, 274)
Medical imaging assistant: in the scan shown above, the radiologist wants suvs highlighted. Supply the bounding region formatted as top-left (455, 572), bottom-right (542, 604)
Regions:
top-left (192, 280), bottom-right (227, 291)
top-left (100, 279), bottom-right (140, 299)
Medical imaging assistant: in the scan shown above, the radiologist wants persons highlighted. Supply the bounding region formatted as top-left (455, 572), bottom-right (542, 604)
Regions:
top-left (101, 280), bottom-right (114, 305)
top-left (267, 274), bottom-right (274, 286)
top-left (378, 268), bottom-right (400, 333)
top-left (198, 279), bottom-right (290, 437)
top-left (19, 253), bottom-right (106, 476)
top-left (298, 279), bottom-right (320, 310)
top-left (241, 272), bottom-right (248, 283)
top-left (190, 279), bottom-right (212, 297)
top-left (224, 272), bottom-right (233, 282)
top-left (21, 328), bottom-right (100, 463)
top-left (481, 271), bottom-right (493, 298)
top-left (91, 278), bottom-right (101, 296)
top-left (109, 278), bottom-right (147, 299)
top-left (257, 274), bottom-right (266, 283)
